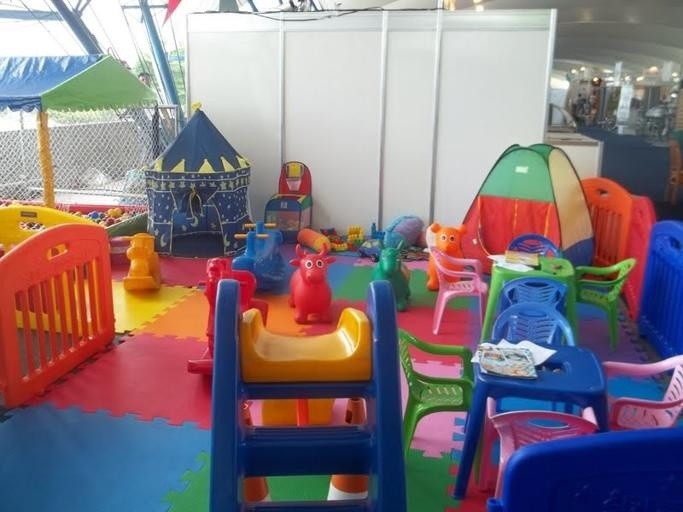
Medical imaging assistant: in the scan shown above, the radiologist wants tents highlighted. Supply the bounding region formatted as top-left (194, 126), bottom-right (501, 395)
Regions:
top-left (458, 143), bottom-right (597, 277)
top-left (144, 104), bottom-right (254, 258)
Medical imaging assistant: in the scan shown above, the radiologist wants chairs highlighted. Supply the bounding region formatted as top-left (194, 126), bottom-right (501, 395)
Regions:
top-left (487, 399), bottom-right (597, 499)
top-left (490, 302), bottom-right (578, 424)
top-left (485, 427), bottom-right (681, 511)
top-left (603, 351), bottom-right (683, 430)
top-left (428, 246), bottom-right (488, 334)
top-left (496, 276), bottom-right (570, 317)
top-left (575, 257), bottom-right (637, 350)
top-left (507, 234), bottom-right (565, 264)
top-left (398, 330), bottom-right (484, 484)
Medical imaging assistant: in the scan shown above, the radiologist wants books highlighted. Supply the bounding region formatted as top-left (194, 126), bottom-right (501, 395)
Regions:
top-left (478, 348), bottom-right (538, 379)
top-left (505, 249), bottom-right (539, 266)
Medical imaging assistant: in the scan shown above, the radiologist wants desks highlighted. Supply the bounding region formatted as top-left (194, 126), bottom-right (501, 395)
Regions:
top-left (479, 257), bottom-right (579, 347)
top-left (453, 342), bottom-right (608, 498)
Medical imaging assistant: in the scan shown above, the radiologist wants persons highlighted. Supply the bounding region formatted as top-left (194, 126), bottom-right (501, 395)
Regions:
top-left (573, 88), bottom-right (601, 123)
top-left (138, 73), bottom-right (150, 87)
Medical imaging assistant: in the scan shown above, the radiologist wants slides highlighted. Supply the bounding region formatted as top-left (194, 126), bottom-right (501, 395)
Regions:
top-left (242, 309), bottom-right (369, 426)
